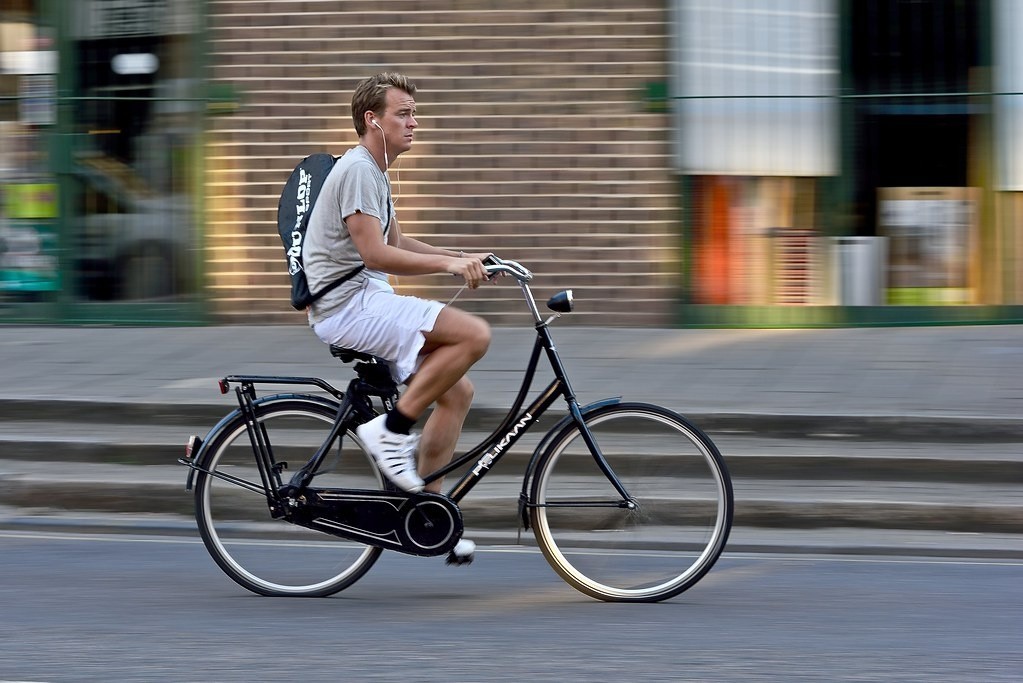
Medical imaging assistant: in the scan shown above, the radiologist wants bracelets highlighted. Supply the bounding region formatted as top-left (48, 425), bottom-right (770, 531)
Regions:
top-left (459, 250), bottom-right (464, 257)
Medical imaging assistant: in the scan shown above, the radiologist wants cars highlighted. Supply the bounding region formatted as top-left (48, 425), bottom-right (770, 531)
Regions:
top-left (70, 149), bottom-right (194, 298)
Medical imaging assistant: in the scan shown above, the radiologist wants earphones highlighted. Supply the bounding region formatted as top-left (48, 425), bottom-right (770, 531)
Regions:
top-left (372, 120), bottom-right (381, 129)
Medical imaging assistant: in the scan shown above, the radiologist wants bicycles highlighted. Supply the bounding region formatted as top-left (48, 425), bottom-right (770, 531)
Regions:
top-left (176, 255), bottom-right (735, 605)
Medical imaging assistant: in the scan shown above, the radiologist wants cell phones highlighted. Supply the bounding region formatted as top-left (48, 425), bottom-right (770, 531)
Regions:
top-left (481, 254), bottom-right (503, 282)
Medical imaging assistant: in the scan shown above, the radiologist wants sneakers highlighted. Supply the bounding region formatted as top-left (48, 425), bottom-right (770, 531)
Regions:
top-left (454, 538), bottom-right (476, 556)
top-left (356, 413), bottom-right (425, 494)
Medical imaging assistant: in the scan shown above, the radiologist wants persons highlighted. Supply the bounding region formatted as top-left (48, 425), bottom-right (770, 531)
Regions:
top-left (301, 73), bottom-right (507, 557)
